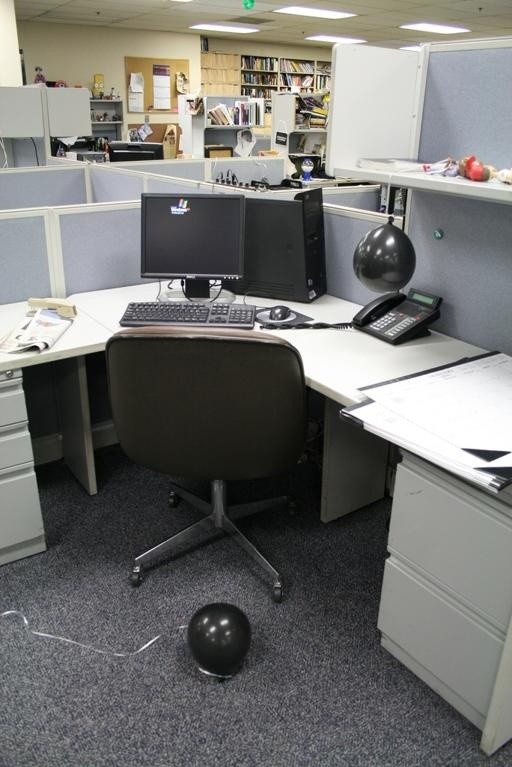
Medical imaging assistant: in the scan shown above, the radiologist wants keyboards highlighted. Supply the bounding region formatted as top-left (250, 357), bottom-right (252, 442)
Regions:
top-left (119, 303), bottom-right (256, 329)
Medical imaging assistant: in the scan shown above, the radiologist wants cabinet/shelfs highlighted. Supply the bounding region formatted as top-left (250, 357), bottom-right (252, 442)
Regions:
top-left (376, 448), bottom-right (511, 753)
top-left (51, 97), bottom-right (127, 151)
top-left (0, 369), bottom-right (49, 566)
top-left (200, 94), bottom-right (266, 157)
top-left (268, 87), bottom-right (330, 179)
top-left (201, 55), bottom-right (332, 114)
top-left (328, 34), bottom-right (512, 206)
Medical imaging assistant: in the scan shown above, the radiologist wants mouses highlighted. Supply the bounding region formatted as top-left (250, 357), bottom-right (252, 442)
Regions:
top-left (269, 305), bottom-right (291, 322)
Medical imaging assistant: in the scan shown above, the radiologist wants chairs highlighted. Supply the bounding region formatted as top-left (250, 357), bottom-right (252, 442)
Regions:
top-left (104, 327), bottom-right (311, 602)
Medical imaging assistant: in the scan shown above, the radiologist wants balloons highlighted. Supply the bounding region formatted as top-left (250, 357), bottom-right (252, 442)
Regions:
top-left (185, 601), bottom-right (253, 678)
top-left (352, 217), bottom-right (417, 294)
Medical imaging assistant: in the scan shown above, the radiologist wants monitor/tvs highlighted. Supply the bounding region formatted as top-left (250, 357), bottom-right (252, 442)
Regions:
top-left (139, 192), bottom-right (245, 303)
top-left (108, 142), bottom-right (163, 161)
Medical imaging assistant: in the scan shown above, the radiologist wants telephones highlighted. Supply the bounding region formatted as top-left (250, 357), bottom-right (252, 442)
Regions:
top-left (350, 288), bottom-right (442, 345)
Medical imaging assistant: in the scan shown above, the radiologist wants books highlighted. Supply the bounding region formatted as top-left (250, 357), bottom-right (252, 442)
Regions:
top-left (207, 55), bottom-right (332, 129)
top-left (0, 305), bottom-right (74, 356)
top-left (339, 349), bottom-right (512, 494)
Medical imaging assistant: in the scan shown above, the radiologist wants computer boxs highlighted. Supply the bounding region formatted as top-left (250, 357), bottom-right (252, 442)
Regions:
top-left (222, 189), bottom-right (327, 304)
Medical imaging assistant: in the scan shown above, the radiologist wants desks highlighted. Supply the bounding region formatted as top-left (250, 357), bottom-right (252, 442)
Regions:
top-left (0, 152), bottom-right (512, 491)
